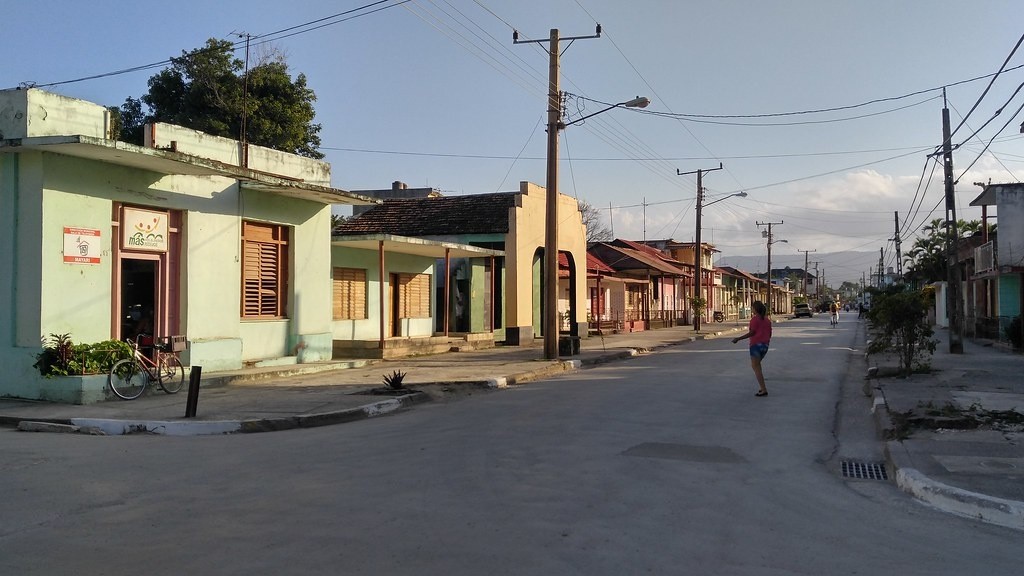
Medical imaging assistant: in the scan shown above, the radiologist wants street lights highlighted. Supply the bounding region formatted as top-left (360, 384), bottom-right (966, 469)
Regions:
top-left (765, 240), bottom-right (788, 317)
top-left (693, 192), bottom-right (748, 330)
top-left (543, 97), bottom-right (652, 361)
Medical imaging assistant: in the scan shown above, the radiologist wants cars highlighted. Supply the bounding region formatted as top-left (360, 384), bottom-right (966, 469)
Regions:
top-left (822, 301), bottom-right (833, 313)
top-left (795, 303), bottom-right (813, 318)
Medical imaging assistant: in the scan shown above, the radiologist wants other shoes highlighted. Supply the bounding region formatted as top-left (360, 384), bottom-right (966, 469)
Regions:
top-left (755, 388), bottom-right (768, 396)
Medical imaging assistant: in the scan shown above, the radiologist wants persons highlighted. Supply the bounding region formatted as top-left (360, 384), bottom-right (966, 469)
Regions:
top-left (814, 300), bottom-right (853, 314)
top-left (731, 301), bottom-right (772, 397)
top-left (829, 301), bottom-right (840, 325)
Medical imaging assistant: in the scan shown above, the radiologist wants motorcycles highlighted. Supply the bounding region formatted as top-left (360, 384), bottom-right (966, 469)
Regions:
top-left (714, 311), bottom-right (725, 323)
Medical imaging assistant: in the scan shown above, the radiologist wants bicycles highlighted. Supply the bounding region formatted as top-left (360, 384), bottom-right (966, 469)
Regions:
top-left (109, 332), bottom-right (186, 400)
top-left (830, 311), bottom-right (839, 328)
